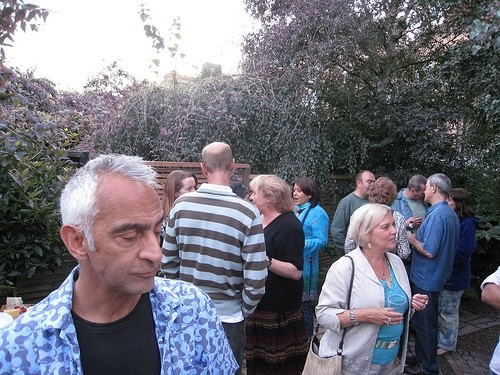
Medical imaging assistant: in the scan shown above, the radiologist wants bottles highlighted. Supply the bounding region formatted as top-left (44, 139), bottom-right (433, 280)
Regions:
top-left (408, 221), bottom-right (414, 248)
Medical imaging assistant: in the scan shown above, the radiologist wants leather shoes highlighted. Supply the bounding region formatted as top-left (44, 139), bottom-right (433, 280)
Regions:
top-left (404, 363), bottom-right (438, 375)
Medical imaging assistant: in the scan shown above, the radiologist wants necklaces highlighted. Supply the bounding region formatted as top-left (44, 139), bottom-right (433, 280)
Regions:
top-left (374, 264), bottom-right (385, 276)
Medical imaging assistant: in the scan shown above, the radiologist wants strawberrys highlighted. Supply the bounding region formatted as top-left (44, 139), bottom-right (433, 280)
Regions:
top-left (14, 306), bottom-right (26, 312)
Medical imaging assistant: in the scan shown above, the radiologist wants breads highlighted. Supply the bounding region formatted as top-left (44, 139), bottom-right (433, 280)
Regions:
top-left (4, 309), bottom-right (20, 317)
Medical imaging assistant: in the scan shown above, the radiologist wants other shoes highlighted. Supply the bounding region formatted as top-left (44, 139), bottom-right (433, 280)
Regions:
top-left (437, 348), bottom-right (452, 355)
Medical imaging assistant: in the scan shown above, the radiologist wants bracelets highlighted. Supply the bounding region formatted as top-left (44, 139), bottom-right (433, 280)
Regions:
top-left (349, 306), bottom-right (358, 327)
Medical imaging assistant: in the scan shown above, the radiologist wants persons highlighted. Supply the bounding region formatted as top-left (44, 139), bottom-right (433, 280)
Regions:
top-left (291, 177), bottom-right (330, 339)
top-left (480, 265), bottom-right (500, 375)
top-left (344, 176), bottom-right (412, 260)
top-left (331, 169), bottom-right (381, 256)
top-left (301, 202), bottom-right (429, 375)
top-left (157, 141), bottom-right (269, 375)
top-left (230, 181), bottom-right (251, 201)
top-left (0, 155), bottom-right (240, 375)
top-left (244, 175), bottom-right (311, 375)
top-left (159, 169), bottom-right (198, 244)
top-left (404, 172), bottom-right (460, 375)
top-left (391, 174), bottom-right (431, 235)
top-left (435, 187), bottom-right (481, 355)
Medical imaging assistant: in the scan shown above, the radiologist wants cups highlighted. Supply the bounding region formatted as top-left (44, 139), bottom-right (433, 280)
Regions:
top-left (7, 297), bottom-right (22, 311)
top-left (387, 294), bottom-right (406, 323)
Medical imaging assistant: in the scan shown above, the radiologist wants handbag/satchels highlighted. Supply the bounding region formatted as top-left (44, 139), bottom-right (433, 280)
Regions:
top-left (301, 335), bottom-right (343, 375)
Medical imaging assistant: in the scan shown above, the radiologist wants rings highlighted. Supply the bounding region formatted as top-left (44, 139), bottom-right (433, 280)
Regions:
top-left (422, 304), bottom-right (426, 309)
top-left (267, 256), bottom-right (272, 268)
top-left (385, 320), bottom-right (390, 325)
top-left (425, 299), bottom-right (427, 304)
top-left (387, 317), bottom-right (391, 322)
